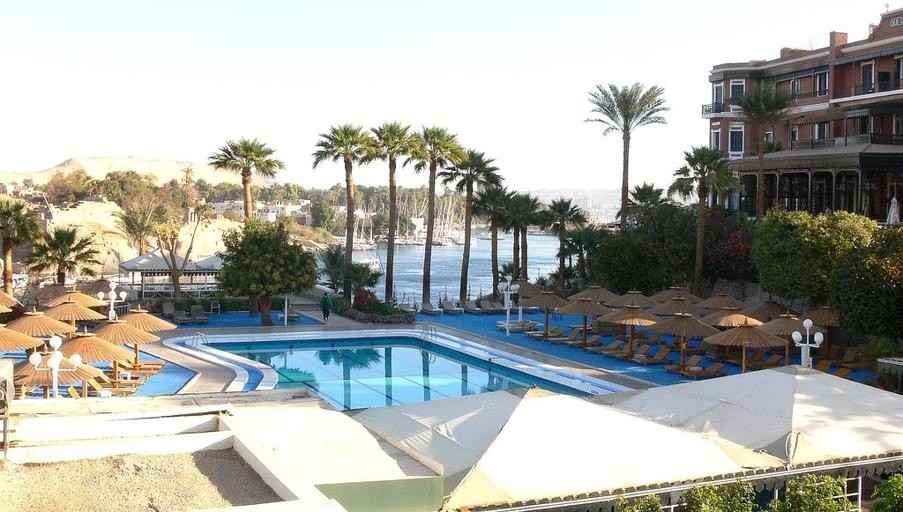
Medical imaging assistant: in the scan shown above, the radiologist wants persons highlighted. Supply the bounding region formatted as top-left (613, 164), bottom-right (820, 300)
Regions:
top-left (318, 291), bottom-right (334, 319)
top-left (824, 205), bottom-right (832, 215)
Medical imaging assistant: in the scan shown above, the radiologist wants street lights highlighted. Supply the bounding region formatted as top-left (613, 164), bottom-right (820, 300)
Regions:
top-left (30, 335), bottom-right (83, 398)
top-left (496, 274), bottom-right (521, 337)
top-left (792, 319), bottom-right (824, 366)
top-left (97, 281), bottom-right (128, 322)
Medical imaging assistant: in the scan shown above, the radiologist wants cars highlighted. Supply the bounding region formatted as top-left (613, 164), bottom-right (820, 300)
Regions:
top-left (0, 273), bottom-right (26, 287)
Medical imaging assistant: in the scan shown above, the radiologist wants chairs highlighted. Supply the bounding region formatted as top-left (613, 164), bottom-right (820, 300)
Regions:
top-left (393, 299), bottom-right (540, 318)
top-left (156, 299), bottom-right (226, 328)
top-left (281, 304), bottom-right (303, 324)
top-left (494, 320), bottom-right (870, 379)
top-left (14, 359), bottom-right (166, 399)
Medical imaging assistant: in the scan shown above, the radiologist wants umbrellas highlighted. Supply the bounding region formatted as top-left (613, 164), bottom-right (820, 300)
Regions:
top-left (885, 195), bottom-right (900, 224)
top-left (0, 289), bottom-right (179, 399)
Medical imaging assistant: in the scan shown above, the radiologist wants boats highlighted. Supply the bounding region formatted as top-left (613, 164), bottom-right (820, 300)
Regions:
top-left (352, 231), bottom-right (506, 251)
